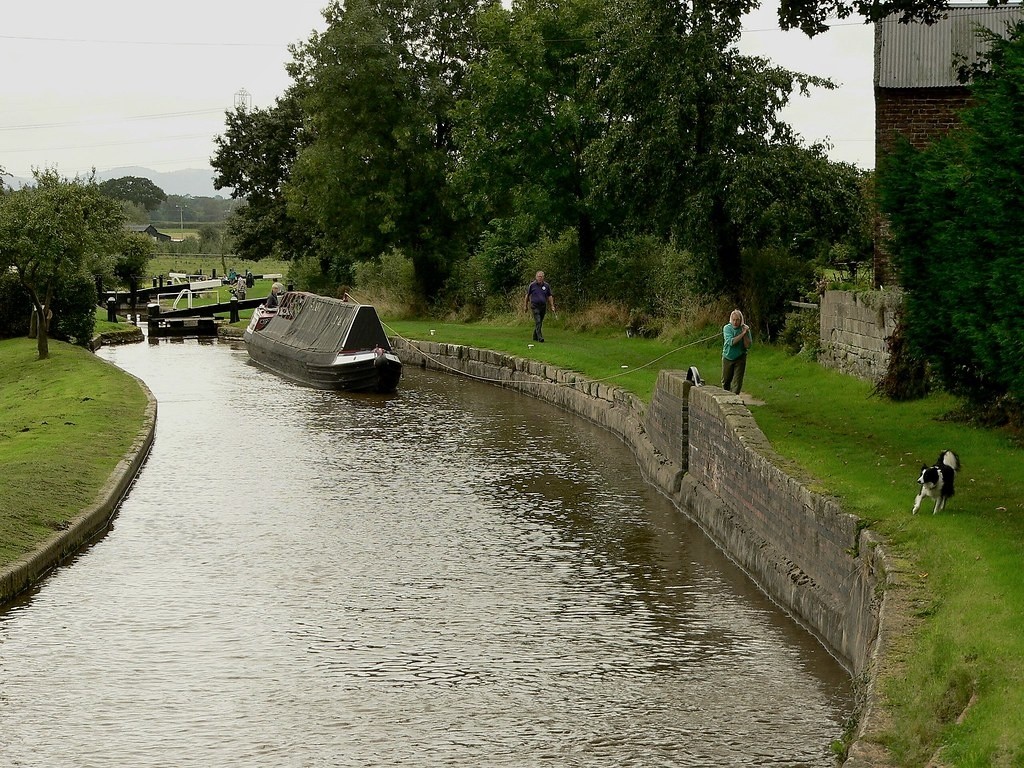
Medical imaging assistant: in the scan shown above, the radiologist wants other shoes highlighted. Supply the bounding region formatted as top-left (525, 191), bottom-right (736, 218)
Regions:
top-left (538, 338), bottom-right (544, 342)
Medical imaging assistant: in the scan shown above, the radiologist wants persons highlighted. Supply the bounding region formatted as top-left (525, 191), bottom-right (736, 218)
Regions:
top-left (271, 278), bottom-right (286, 295)
top-left (267, 284), bottom-right (279, 308)
top-left (525, 271), bottom-right (555, 342)
top-left (224, 268), bottom-right (254, 300)
top-left (722, 310), bottom-right (752, 396)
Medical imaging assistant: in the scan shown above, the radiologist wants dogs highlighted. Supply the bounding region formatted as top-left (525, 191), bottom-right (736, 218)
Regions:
top-left (911, 447), bottom-right (962, 517)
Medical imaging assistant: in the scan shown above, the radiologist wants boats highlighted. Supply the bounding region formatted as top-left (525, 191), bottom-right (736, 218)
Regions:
top-left (244, 290), bottom-right (403, 394)
